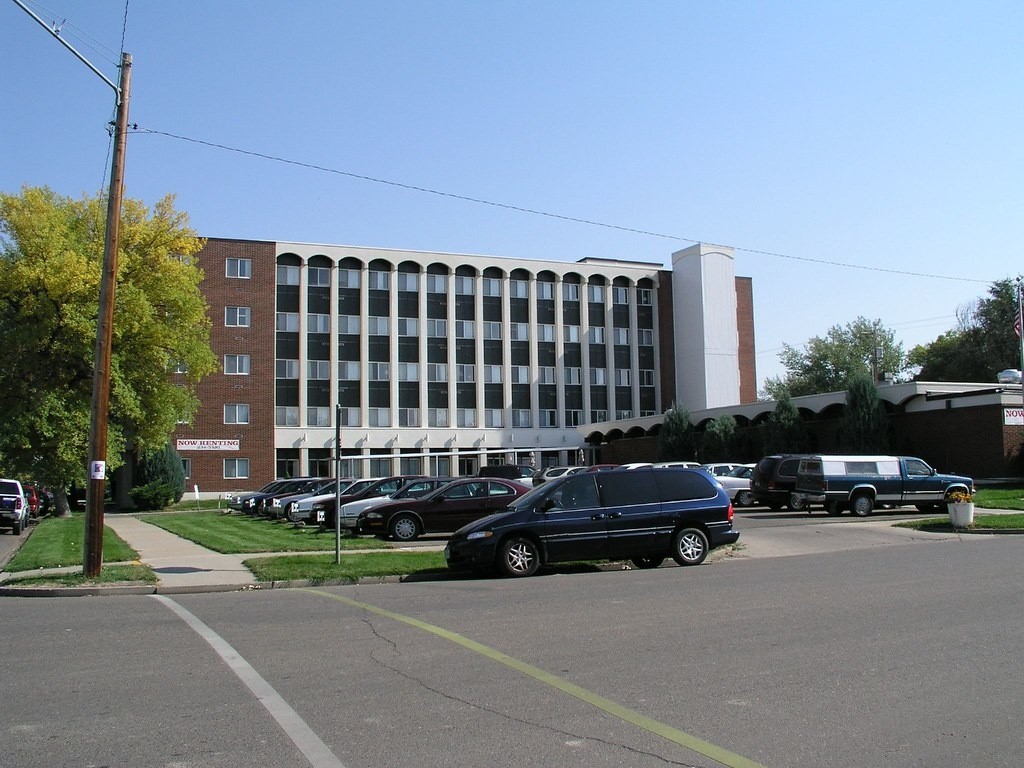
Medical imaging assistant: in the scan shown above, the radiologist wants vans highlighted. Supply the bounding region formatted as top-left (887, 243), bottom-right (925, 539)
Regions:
top-left (443, 469), bottom-right (740, 578)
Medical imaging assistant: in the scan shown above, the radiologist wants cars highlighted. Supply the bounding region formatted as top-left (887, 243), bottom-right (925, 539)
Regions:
top-left (355, 476), bottom-right (562, 542)
top-left (340, 477), bottom-right (433, 528)
top-left (226, 475), bottom-right (396, 527)
top-left (0, 479), bottom-right (50, 535)
top-left (480, 447), bottom-right (758, 508)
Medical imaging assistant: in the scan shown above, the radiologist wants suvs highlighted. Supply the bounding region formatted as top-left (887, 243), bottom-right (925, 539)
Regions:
top-left (749, 455), bottom-right (813, 512)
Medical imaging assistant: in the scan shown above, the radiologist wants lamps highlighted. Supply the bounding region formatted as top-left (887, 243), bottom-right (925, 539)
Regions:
top-left (537, 435), bottom-right (540, 442)
top-left (482, 434), bottom-right (486, 441)
top-left (299, 433), bottom-right (307, 447)
top-left (511, 433), bottom-right (514, 441)
top-left (454, 433), bottom-right (458, 441)
top-left (564, 435), bottom-right (567, 441)
top-left (424, 433), bottom-right (429, 442)
top-left (395, 434), bottom-right (399, 442)
top-left (363, 433), bottom-right (369, 443)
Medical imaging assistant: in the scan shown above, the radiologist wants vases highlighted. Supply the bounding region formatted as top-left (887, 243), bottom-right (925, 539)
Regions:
top-left (947, 502), bottom-right (975, 526)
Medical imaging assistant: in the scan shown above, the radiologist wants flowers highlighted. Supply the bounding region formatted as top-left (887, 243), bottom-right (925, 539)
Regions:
top-left (944, 491), bottom-right (972, 503)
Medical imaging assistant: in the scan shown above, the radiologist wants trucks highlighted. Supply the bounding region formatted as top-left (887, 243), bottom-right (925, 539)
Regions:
top-left (790, 456), bottom-right (977, 519)
